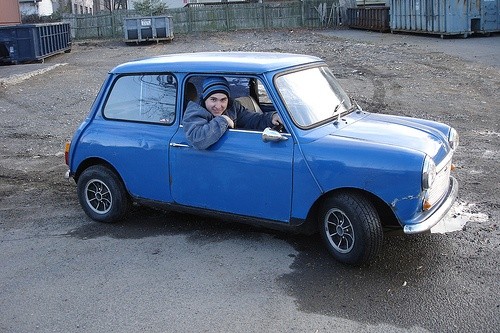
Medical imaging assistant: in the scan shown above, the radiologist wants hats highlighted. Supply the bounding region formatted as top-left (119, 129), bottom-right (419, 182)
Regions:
top-left (202, 76), bottom-right (231, 101)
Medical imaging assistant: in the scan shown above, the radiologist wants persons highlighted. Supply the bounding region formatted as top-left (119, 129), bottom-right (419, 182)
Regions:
top-left (181, 75), bottom-right (285, 152)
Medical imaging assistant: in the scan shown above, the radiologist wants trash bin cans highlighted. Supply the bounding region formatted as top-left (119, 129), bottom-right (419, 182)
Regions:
top-left (470, 17), bottom-right (481, 32)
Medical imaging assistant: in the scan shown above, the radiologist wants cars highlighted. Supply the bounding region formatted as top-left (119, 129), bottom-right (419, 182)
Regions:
top-left (67, 51), bottom-right (460, 268)
